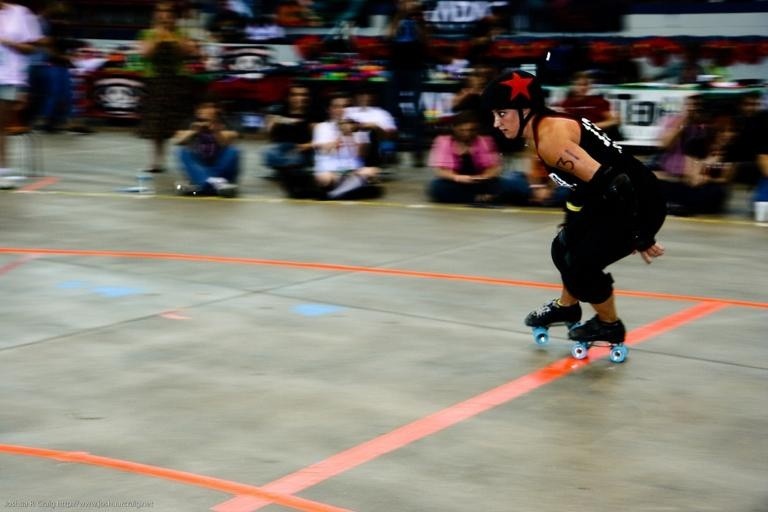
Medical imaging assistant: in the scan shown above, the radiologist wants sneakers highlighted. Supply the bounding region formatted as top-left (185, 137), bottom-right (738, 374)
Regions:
top-left (136, 164), bottom-right (167, 179)
top-left (207, 177), bottom-right (239, 193)
top-left (173, 179), bottom-right (203, 194)
top-left (0, 167), bottom-right (29, 189)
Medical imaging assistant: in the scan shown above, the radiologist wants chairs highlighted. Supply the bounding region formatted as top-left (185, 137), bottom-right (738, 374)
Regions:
top-left (3, 63), bottom-right (72, 174)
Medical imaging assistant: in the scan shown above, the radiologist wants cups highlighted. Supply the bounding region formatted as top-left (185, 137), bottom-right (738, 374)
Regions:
top-left (754, 201), bottom-right (768, 222)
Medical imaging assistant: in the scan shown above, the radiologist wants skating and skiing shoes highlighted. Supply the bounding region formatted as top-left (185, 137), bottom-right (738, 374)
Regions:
top-left (524, 298), bottom-right (581, 345)
top-left (567, 313), bottom-right (630, 364)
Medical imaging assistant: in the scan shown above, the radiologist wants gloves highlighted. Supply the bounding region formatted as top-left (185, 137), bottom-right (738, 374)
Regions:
top-left (625, 225), bottom-right (656, 252)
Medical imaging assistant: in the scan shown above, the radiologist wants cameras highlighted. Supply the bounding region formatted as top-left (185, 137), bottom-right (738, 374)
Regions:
top-left (186, 119), bottom-right (210, 132)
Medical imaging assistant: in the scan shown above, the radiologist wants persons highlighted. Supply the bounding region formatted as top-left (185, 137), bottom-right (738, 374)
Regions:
top-left (0, 0), bottom-right (767, 219)
top-left (482, 69), bottom-right (668, 343)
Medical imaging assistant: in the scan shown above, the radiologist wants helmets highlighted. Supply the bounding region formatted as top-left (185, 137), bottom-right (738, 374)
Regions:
top-left (480, 69), bottom-right (544, 111)
